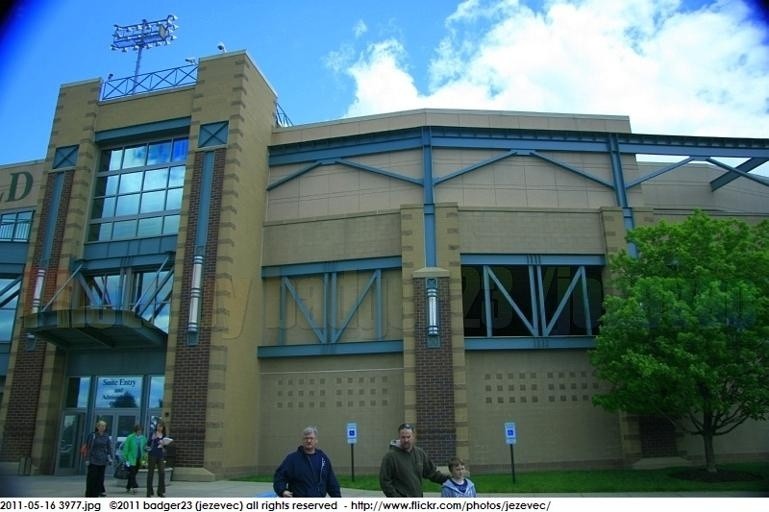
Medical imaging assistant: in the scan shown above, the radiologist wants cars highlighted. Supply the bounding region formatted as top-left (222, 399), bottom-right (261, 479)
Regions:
top-left (59, 436), bottom-right (128, 467)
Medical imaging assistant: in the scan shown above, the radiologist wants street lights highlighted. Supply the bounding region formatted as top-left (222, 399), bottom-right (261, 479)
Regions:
top-left (110, 14), bottom-right (178, 96)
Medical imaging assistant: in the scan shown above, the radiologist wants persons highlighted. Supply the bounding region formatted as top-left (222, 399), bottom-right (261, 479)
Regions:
top-left (85, 420), bottom-right (113, 497)
top-left (147, 420), bottom-right (166, 497)
top-left (121, 425), bottom-right (148, 493)
top-left (441, 457), bottom-right (477, 497)
top-left (380, 424), bottom-right (471, 497)
top-left (273, 427), bottom-right (341, 497)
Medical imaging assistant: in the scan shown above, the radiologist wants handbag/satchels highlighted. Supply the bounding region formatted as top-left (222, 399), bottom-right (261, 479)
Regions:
top-left (81, 444), bottom-right (89, 457)
top-left (144, 444), bottom-right (152, 452)
top-left (114, 463), bottom-right (132, 479)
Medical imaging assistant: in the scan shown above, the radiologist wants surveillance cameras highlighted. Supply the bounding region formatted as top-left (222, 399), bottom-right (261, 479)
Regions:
top-left (217, 42), bottom-right (227, 55)
top-left (183, 58), bottom-right (196, 64)
top-left (106, 73), bottom-right (115, 81)
